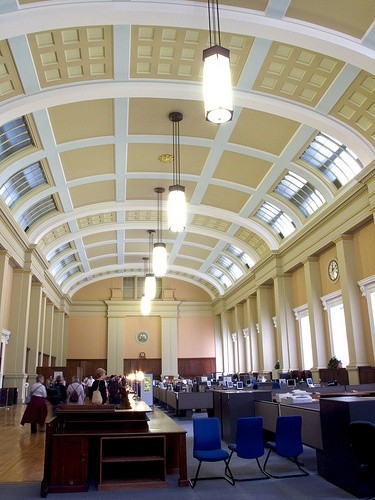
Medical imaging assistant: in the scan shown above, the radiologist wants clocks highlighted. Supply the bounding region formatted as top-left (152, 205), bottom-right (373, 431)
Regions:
top-left (140, 352), bottom-right (145, 357)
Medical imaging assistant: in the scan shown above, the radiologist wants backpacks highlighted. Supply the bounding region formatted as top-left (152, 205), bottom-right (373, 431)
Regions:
top-left (68, 384), bottom-right (82, 403)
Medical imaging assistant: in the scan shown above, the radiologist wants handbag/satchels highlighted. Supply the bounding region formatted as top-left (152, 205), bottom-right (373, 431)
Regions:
top-left (25, 392), bottom-right (32, 404)
top-left (91, 380), bottom-right (103, 404)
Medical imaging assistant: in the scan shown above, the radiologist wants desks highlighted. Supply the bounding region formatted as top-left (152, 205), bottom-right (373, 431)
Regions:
top-left (39, 380), bottom-right (375, 500)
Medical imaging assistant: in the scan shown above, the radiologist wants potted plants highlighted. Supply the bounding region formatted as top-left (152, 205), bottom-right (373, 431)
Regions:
top-left (327, 357), bottom-right (342, 369)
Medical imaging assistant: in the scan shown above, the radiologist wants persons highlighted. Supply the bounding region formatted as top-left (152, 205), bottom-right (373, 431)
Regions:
top-left (30, 375), bottom-right (47, 433)
top-left (46, 367), bottom-right (130, 405)
top-left (272, 363), bottom-right (283, 382)
top-left (328, 358), bottom-right (338, 368)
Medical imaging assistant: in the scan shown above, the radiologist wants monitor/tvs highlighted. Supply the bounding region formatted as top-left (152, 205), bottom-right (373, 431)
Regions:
top-left (184, 376), bottom-right (313, 387)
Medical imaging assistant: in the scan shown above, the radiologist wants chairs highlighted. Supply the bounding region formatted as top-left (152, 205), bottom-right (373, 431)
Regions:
top-left (263, 415), bottom-right (310, 479)
top-left (224, 416), bottom-right (271, 482)
top-left (187, 417), bottom-right (235, 489)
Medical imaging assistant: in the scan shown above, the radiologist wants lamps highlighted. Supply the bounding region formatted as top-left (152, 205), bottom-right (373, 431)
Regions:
top-left (166, 111), bottom-right (187, 233)
top-left (140, 257), bottom-right (151, 316)
top-left (143, 230), bottom-right (156, 301)
top-left (152, 187), bottom-right (167, 276)
top-left (201, 0), bottom-right (234, 124)
